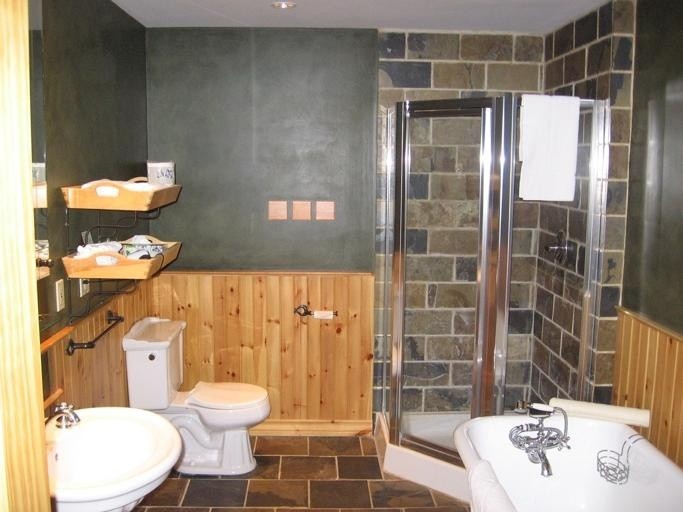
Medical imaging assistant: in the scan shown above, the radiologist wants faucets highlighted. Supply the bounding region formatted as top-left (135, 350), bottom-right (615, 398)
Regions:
top-left (45, 409), bottom-right (81, 433)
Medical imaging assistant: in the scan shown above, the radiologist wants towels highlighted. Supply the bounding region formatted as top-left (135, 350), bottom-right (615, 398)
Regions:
top-left (518, 92), bottom-right (580, 204)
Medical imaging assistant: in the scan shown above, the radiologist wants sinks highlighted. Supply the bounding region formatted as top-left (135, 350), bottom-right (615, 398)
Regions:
top-left (45, 407), bottom-right (184, 512)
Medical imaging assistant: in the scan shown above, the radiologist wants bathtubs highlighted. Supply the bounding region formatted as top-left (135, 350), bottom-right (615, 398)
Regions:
top-left (453, 414), bottom-right (683, 512)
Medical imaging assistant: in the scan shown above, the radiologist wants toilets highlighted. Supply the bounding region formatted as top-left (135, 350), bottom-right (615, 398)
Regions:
top-left (121, 316), bottom-right (271, 476)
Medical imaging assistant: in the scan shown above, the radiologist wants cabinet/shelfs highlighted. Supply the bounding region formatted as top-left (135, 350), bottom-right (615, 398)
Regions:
top-left (59, 176), bottom-right (182, 327)
